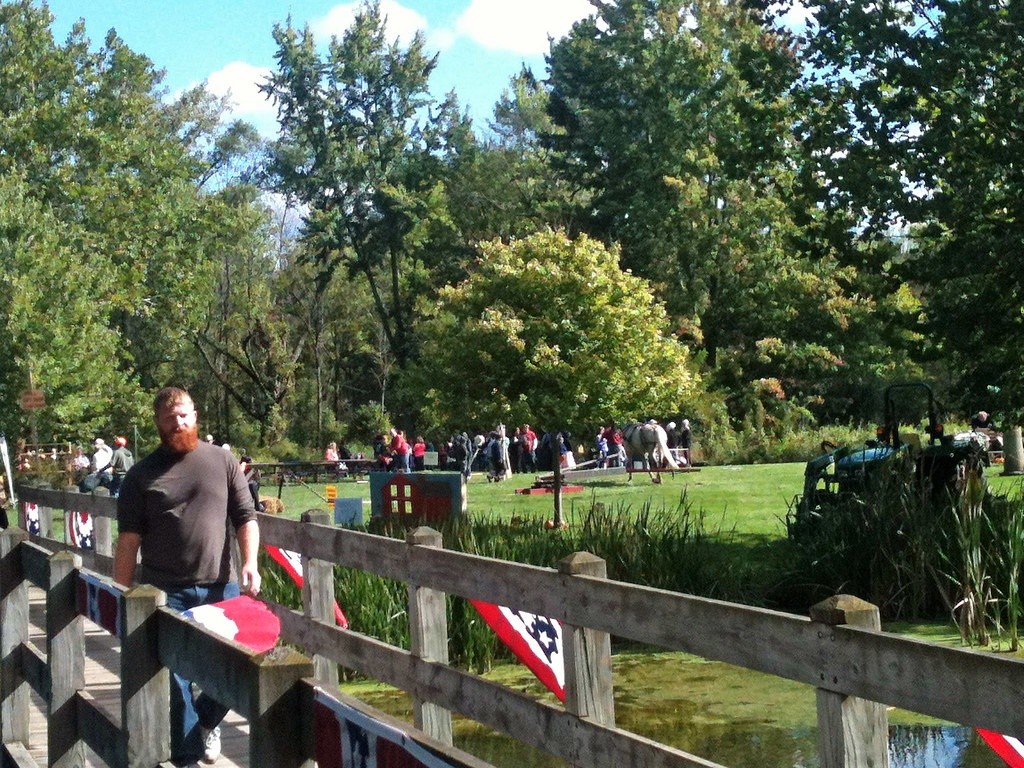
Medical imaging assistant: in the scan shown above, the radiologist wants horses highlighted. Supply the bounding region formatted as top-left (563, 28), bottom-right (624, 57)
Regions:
top-left (620, 419), bottom-right (680, 485)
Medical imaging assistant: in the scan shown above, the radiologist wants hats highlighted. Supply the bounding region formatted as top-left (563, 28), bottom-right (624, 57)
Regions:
top-left (95, 437), bottom-right (105, 445)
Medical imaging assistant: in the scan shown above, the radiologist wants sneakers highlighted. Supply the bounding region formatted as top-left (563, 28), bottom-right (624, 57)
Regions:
top-left (181, 724), bottom-right (221, 768)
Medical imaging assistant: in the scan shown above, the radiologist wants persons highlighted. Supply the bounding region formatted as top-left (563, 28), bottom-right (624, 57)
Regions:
top-left (0, 436), bottom-right (136, 529)
top-left (113, 388), bottom-right (262, 768)
top-left (325, 418), bottom-right (692, 484)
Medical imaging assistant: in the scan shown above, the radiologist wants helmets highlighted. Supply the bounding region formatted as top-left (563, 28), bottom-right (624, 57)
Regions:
top-left (113, 437), bottom-right (127, 446)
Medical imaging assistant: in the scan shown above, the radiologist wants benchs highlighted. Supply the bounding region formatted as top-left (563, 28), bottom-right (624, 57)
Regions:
top-left (253, 456), bottom-right (380, 485)
